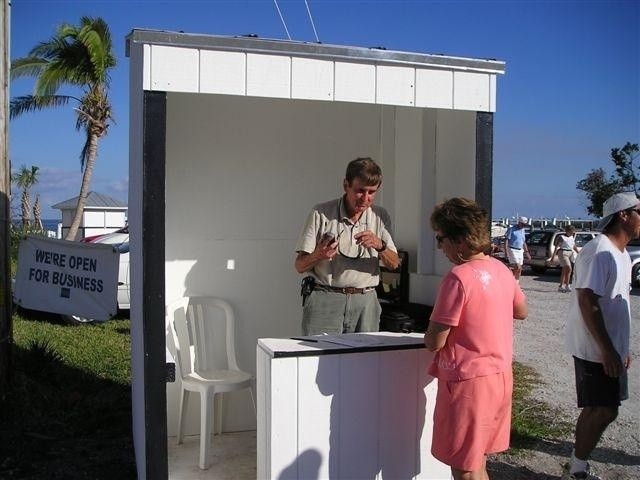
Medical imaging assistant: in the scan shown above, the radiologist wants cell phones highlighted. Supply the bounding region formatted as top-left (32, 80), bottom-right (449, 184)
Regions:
top-left (325, 232), bottom-right (335, 246)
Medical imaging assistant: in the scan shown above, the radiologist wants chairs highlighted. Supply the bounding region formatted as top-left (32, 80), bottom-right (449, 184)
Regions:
top-left (168, 295), bottom-right (258, 471)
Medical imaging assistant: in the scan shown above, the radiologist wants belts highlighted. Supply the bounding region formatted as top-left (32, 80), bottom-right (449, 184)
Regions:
top-left (315, 280), bottom-right (375, 294)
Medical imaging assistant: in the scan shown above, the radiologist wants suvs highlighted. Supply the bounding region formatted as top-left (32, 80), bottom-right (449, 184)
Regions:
top-left (524, 228), bottom-right (578, 275)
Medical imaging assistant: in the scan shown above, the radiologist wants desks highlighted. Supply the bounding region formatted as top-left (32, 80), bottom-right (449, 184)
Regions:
top-left (378, 300), bottom-right (435, 334)
top-left (256, 331), bottom-right (456, 480)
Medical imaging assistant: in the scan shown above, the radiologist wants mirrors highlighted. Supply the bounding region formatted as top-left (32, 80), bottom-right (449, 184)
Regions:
top-left (374, 249), bottom-right (410, 305)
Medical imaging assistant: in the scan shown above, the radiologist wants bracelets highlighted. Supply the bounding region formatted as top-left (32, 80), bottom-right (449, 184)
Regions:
top-left (375, 239), bottom-right (387, 253)
top-left (526, 250), bottom-right (529, 252)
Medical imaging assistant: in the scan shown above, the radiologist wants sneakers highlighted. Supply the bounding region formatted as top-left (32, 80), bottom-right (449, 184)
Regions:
top-left (561, 462), bottom-right (602, 480)
top-left (559, 284), bottom-right (572, 292)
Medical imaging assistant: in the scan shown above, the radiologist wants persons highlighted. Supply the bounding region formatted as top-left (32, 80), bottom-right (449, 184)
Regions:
top-left (293, 158), bottom-right (402, 334)
top-left (504, 215), bottom-right (532, 284)
top-left (425, 196), bottom-right (527, 479)
top-left (561, 192), bottom-right (640, 480)
top-left (549, 225), bottom-right (578, 292)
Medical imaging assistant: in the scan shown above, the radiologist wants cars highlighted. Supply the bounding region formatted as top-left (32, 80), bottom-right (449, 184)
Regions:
top-left (61, 225), bottom-right (130, 324)
top-left (570, 231), bottom-right (603, 264)
top-left (626, 238), bottom-right (640, 288)
top-left (494, 228), bottom-right (530, 262)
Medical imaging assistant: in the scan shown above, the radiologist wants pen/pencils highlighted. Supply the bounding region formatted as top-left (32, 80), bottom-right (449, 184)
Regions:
top-left (291, 338), bottom-right (317, 343)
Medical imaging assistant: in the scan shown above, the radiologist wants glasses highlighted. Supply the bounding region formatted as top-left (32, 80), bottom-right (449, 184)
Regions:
top-left (436, 234), bottom-right (452, 243)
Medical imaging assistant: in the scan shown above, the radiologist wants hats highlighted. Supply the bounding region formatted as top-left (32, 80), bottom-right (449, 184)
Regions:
top-left (596, 192), bottom-right (639, 231)
top-left (519, 216), bottom-right (531, 227)
